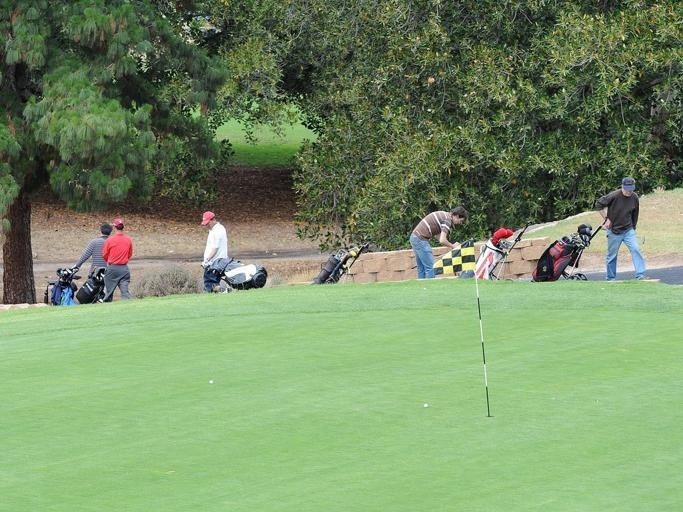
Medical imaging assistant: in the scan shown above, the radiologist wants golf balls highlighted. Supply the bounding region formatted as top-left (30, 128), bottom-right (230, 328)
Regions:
top-left (209, 381), bottom-right (213, 384)
top-left (424, 404), bottom-right (429, 407)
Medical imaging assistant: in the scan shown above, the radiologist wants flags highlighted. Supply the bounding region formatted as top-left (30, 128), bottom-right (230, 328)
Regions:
top-left (432, 239), bottom-right (478, 275)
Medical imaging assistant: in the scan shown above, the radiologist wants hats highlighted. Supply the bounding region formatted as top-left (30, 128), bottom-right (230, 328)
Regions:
top-left (622, 177), bottom-right (635, 191)
top-left (201, 211), bottom-right (215, 225)
top-left (109, 218), bottom-right (123, 226)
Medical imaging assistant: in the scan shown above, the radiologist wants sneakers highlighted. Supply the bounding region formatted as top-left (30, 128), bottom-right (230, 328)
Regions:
top-left (637, 276), bottom-right (651, 280)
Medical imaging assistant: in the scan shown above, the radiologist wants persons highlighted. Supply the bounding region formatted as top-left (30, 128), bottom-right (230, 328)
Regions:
top-left (100, 218), bottom-right (132, 300)
top-left (593, 177), bottom-right (651, 280)
top-left (408, 205), bottom-right (466, 278)
top-left (199, 210), bottom-right (228, 292)
top-left (72, 222), bottom-right (112, 276)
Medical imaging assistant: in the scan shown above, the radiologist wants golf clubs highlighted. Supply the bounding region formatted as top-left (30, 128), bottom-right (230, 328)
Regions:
top-left (335, 244), bottom-right (359, 262)
top-left (496, 239), bottom-right (513, 249)
top-left (56, 265), bottom-right (78, 284)
top-left (562, 233), bottom-right (590, 249)
